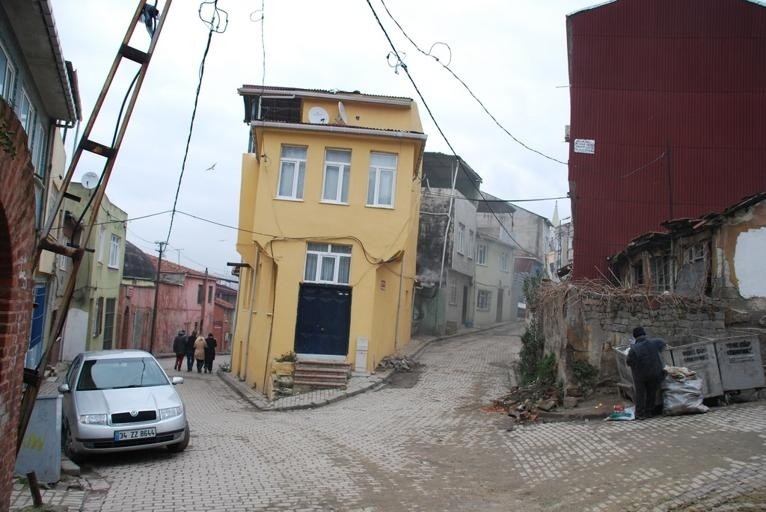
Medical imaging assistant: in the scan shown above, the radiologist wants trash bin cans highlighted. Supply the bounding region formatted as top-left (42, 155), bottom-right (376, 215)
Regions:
top-left (17, 397), bottom-right (62, 484)
top-left (611, 329), bottom-right (764, 405)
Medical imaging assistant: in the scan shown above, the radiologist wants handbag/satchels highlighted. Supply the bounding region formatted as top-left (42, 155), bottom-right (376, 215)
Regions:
top-left (636, 356), bottom-right (656, 381)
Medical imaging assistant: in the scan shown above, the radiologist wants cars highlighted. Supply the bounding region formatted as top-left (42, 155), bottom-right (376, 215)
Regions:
top-left (57, 346), bottom-right (190, 464)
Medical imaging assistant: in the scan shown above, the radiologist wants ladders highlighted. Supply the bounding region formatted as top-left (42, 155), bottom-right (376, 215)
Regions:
top-left (16, 0), bottom-right (172, 454)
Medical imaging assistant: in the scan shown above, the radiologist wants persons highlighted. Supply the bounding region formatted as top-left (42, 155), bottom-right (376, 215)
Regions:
top-left (173, 330), bottom-right (217, 373)
top-left (626, 327), bottom-right (668, 420)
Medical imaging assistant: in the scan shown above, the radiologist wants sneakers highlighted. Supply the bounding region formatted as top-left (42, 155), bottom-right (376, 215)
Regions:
top-left (636, 408), bottom-right (655, 419)
